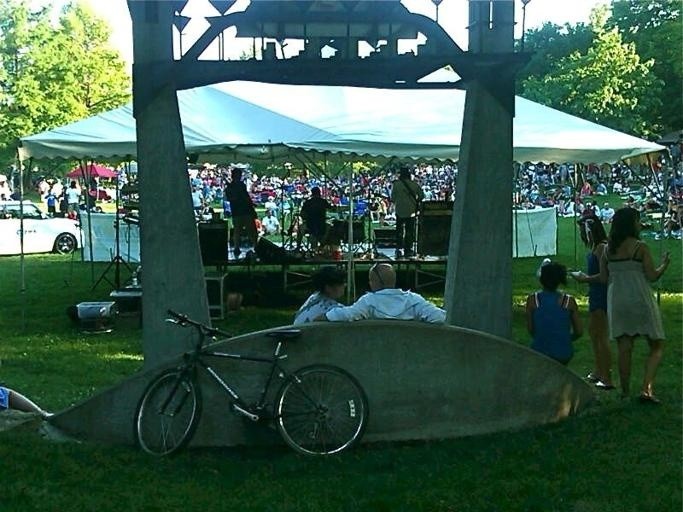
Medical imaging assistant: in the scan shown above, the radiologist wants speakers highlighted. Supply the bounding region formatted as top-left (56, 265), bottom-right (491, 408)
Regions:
top-left (197, 221), bottom-right (230, 268)
top-left (417, 199), bottom-right (453, 258)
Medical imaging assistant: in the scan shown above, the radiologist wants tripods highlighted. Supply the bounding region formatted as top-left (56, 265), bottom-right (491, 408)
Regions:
top-left (353, 199), bottom-right (379, 258)
top-left (87, 211), bottom-right (134, 291)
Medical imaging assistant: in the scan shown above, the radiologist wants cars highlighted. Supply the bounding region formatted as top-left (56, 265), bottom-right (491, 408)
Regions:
top-left (0, 200), bottom-right (86, 255)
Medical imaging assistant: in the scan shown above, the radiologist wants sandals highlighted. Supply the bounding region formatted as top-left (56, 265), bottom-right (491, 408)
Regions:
top-left (640, 389), bottom-right (660, 404)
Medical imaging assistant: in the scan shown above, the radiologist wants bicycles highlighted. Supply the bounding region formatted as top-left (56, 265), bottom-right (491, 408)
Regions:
top-left (283, 231), bottom-right (317, 259)
top-left (134, 309), bottom-right (368, 460)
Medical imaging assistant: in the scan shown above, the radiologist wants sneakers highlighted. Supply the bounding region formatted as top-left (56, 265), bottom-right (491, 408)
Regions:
top-left (586, 371), bottom-right (616, 390)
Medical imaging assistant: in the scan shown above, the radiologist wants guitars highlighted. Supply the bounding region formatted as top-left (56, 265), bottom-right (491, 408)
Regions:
top-left (223, 175), bottom-right (258, 220)
top-left (374, 193), bottom-right (423, 211)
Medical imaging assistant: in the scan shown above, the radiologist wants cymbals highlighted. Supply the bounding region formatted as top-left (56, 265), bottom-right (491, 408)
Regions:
top-left (284, 206), bottom-right (300, 211)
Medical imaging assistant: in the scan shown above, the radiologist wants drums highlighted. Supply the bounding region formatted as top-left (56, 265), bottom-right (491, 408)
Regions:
top-left (332, 218), bottom-right (344, 239)
top-left (345, 219), bottom-right (365, 243)
top-left (328, 204), bottom-right (350, 211)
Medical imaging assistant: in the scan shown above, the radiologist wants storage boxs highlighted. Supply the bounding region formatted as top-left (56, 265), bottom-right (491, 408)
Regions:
top-left (110, 289), bottom-right (142, 312)
top-left (76, 302), bottom-right (116, 321)
top-left (204, 272), bottom-right (229, 320)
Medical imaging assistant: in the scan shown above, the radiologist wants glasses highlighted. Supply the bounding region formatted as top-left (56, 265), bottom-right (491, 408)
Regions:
top-left (370, 262), bottom-right (384, 285)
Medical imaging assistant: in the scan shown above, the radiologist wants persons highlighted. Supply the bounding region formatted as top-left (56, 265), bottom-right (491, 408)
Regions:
top-left (292, 266), bottom-right (348, 326)
top-left (313, 261), bottom-right (448, 325)
top-left (292, 187), bottom-right (336, 252)
top-left (600, 207), bottom-right (672, 404)
top-left (523, 259), bottom-right (585, 365)
top-left (1, 160), bottom-right (108, 219)
top-left (568, 212), bottom-right (612, 390)
top-left (224, 167), bottom-right (260, 261)
top-left (115, 143), bottom-right (682, 240)
top-left (391, 167), bottom-right (426, 258)
